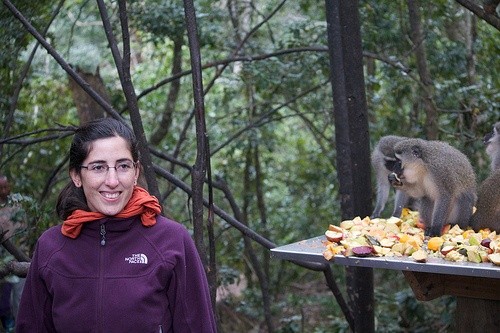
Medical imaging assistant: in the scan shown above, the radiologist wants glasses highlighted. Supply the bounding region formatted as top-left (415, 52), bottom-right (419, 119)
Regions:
top-left (80, 159), bottom-right (135, 172)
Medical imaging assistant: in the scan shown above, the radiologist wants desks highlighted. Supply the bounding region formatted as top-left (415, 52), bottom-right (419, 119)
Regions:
top-left (269, 235), bottom-right (500, 333)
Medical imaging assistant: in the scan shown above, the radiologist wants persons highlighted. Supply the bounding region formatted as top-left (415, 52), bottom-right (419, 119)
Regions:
top-left (0, 175), bottom-right (32, 333)
top-left (12, 119), bottom-right (218, 333)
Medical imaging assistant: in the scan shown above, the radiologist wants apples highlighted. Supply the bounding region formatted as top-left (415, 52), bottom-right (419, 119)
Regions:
top-left (324, 206), bottom-right (500, 268)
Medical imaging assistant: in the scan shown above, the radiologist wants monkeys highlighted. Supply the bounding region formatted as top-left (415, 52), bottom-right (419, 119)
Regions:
top-left (468, 120), bottom-right (500, 235)
top-left (369, 123), bottom-right (479, 240)
